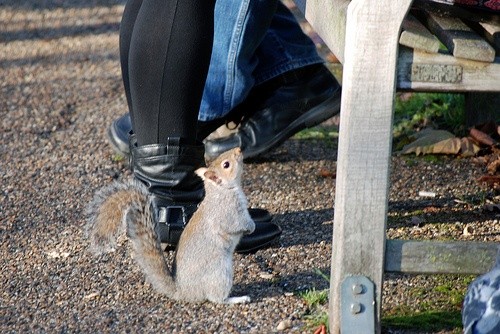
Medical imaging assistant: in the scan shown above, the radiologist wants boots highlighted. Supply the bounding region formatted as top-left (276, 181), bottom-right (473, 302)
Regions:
top-left (131, 141), bottom-right (283, 254)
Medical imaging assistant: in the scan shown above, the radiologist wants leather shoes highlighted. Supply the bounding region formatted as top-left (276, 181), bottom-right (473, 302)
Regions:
top-left (206, 63), bottom-right (342, 161)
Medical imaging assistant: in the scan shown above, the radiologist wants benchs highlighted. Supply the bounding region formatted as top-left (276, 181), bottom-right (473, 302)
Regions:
top-left (295, 0), bottom-right (499, 334)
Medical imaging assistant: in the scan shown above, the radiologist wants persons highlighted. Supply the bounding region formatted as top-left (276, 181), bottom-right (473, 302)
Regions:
top-left (104, 0), bottom-right (342, 164)
top-left (118, 0), bottom-right (284, 255)
